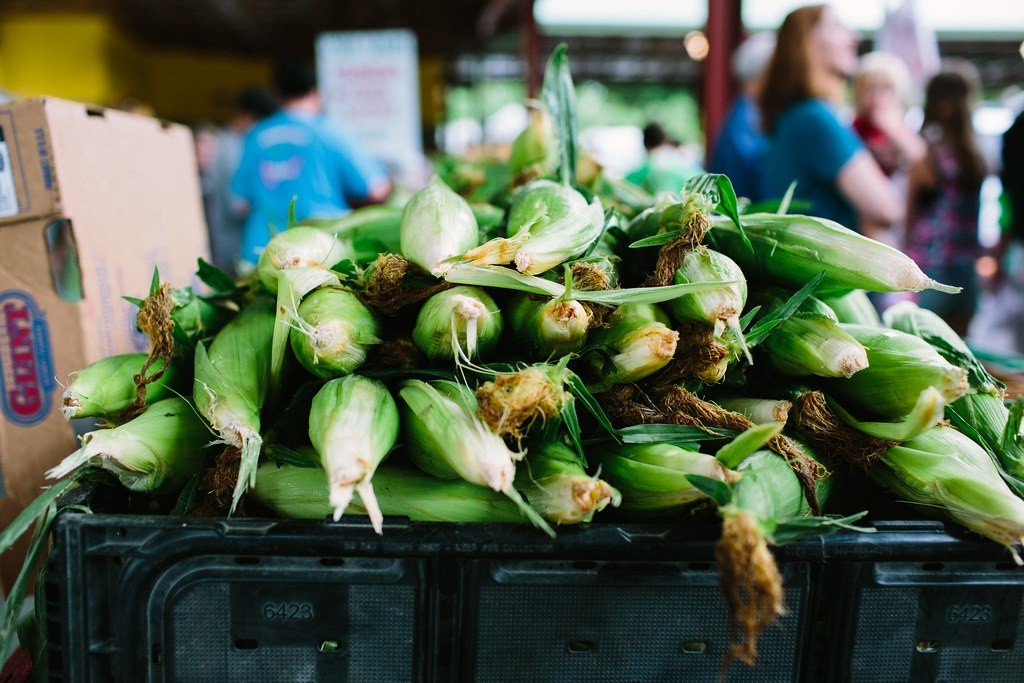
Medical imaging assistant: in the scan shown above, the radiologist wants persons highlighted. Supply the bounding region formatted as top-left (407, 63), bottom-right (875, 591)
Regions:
top-left (645, 3), bottom-right (1023, 348)
top-left (170, 67), bottom-right (381, 278)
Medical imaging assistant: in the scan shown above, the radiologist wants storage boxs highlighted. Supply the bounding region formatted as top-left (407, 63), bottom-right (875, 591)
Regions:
top-left (0, 95), bottom-right (214, 588)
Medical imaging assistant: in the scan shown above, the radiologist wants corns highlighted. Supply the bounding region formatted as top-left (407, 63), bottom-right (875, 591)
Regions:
top-left (39, 175), bottom-right (1022, 683)
